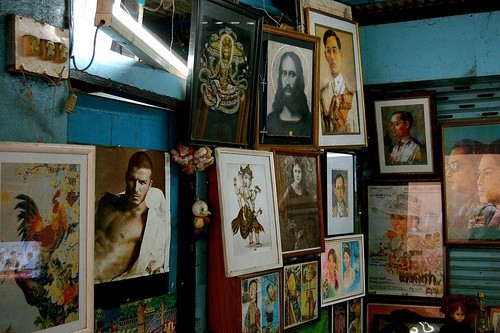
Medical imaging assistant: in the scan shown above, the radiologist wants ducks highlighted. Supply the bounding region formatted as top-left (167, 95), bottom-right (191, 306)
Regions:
top-left (192, 200), bottom-right (211, 233)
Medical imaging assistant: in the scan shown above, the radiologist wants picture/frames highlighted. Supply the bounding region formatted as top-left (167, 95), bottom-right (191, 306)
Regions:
top-left (0, 140), bottom-right (98, 333)
top-left (185, 0), bottom-right (500, 333)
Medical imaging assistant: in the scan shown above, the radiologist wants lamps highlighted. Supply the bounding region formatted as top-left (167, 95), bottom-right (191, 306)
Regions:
top-left (93, 0), bottom-right (188, 78)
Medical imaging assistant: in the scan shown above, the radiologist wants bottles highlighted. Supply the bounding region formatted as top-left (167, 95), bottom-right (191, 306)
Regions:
top-left (474, 292), bottom-right (490, 333)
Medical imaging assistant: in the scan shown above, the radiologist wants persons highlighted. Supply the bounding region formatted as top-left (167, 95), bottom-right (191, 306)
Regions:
top-left (446, 139), bottom-right (500, 227)
top-left (332, 174), bottom-right (348, 217)
top-left (439, 294), bottom-right (479, 333)
top-left (319, 29), bottom-right (360, 135)
top-left (95, 152), bottom-right (170, 285)
top-left (279, 159), bottom-right (311, 213)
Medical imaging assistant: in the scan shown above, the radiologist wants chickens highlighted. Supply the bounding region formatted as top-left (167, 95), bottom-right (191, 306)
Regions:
top-left (14, 189), bottom-right (68, 269)
